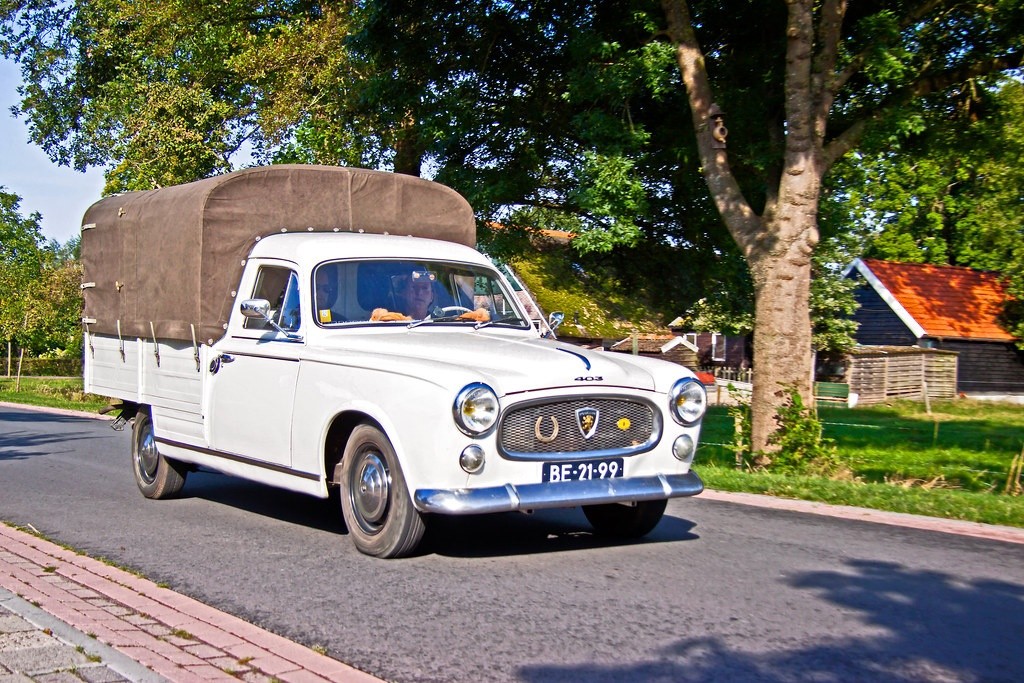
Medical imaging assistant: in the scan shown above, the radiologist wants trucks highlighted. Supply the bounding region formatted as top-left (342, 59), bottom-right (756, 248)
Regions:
top-left (81, 162), bottom-right (709, 562)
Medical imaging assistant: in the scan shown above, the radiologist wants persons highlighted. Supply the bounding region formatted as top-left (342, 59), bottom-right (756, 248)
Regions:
top-left (399, 269), bottom-right (445, 321)
top-left (262, 268), bottom-right (349, 332)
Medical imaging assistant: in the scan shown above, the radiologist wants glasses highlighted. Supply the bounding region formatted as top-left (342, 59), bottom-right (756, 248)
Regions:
top-left (316, 284), bottom-right (332, 293)
